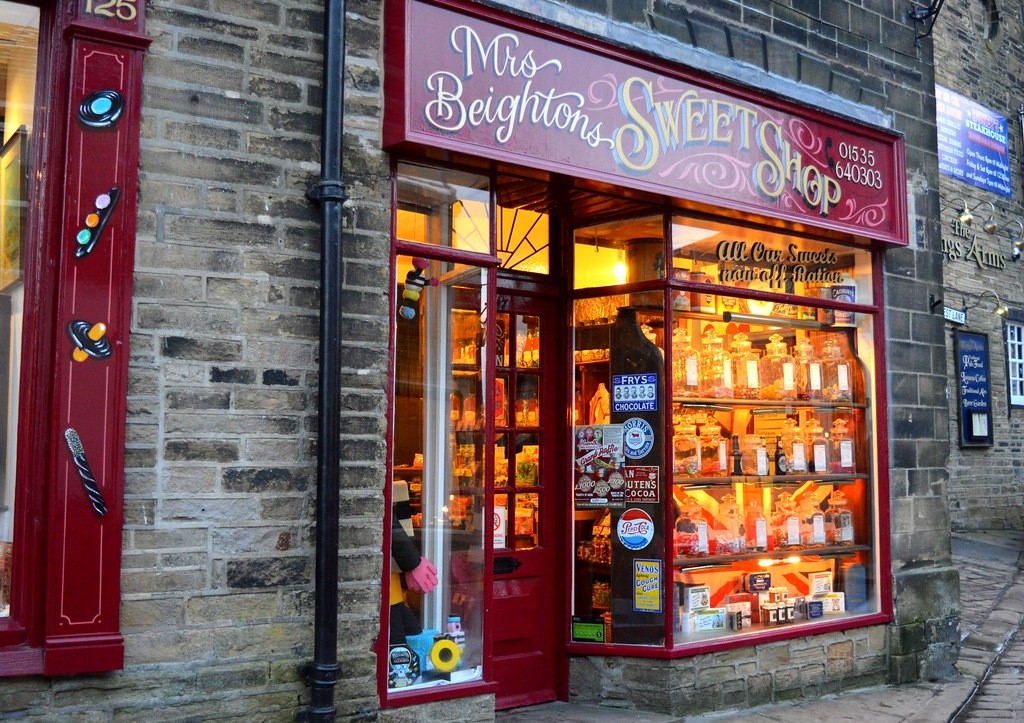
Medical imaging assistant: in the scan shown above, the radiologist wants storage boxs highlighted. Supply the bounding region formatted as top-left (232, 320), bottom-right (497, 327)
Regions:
top-left (679, 556), bottom-right (866, 634)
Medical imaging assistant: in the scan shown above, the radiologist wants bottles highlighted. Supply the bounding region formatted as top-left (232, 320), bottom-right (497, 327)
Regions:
top-left (506, 329), bottom-right (540, 366)
top-left (728, 435), bottom-right (743, 476)
top-left (454, 337), bottom-right (475, 364)
top-left (608, 535), bottom-right (611, 564)
top-left (592, 534), bottom-right (600, 563)
top-left (760, 435), bottom-right (770, 475)
top-left (450, 390), bottom-right (475, 423)
top-left (598, 535), bottom-right (607, 564)
top-left (775, 436), bottom-right (787, 476)
top-left (517, 391), bottom-right (538, 424)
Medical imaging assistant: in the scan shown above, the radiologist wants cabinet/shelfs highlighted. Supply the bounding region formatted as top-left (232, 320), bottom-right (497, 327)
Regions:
top-left (670, 308), bottom-right (879, 646)
top-left (404, 357), bottom-right (608, 535)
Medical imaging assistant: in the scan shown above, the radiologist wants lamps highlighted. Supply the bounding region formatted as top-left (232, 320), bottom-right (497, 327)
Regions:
top-left (998, 220), bottom-right (1023, 262)
top-left (940, 197), bottom-right (973, 227)
top-left (969, 201), bottom-right (997, 235)
